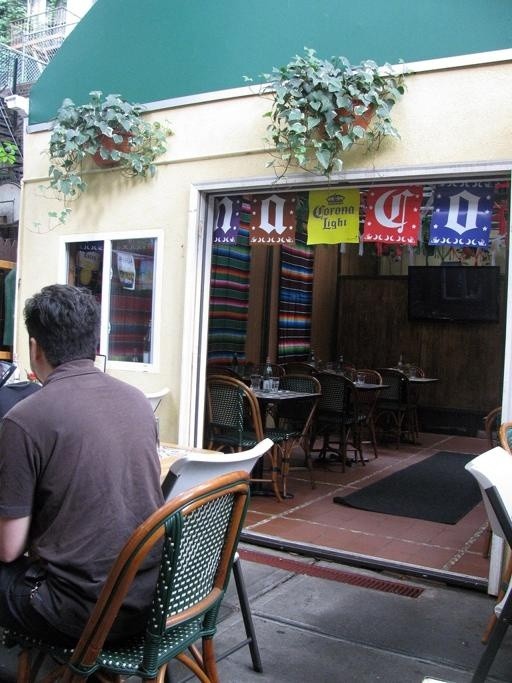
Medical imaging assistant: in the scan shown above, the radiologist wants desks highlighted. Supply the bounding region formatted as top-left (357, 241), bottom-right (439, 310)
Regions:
top-left (146, 439), bottom-right (226, 476)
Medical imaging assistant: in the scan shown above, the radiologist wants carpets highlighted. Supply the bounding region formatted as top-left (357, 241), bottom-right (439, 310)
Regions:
top-left (333, 450), bottom-right (483, 525)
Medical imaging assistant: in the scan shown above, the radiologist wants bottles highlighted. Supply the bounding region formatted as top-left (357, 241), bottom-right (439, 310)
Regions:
top-left (12, 353), bottom-right (20, 380)
top-left (264, 356), bottom-right (272, 393)
top-left (230, 351), bottom-right (240, 375)
top-left (397, 351), bottom-right (403, 371)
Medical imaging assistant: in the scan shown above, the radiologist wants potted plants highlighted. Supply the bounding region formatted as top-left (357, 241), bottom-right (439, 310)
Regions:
top-left (240, 45), bottom-right (413, 194)
top-left (25, 87), bottom-right (175, 237)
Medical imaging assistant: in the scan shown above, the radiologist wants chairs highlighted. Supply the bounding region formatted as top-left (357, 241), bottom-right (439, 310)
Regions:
top-left (1, 471), bottom-right (253, 683)
top-left (205, 360), bottom-right (439, 504)
top-left (153, 437), bottom-right (275, 675)
top-left (464, 446), bottom-right (511, 682)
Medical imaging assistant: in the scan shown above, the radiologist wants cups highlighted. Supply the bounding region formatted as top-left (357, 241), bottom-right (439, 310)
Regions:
top-left (357, 372), bottom-right (366, 384)
top-left (409, 366), bottom-right (417, 379)
top-left (248, 367), bottom-right (260, 390)
top-left (270, 376), bottom-right (280, 393)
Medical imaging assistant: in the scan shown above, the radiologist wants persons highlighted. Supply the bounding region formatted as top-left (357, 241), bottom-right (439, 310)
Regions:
top-left (0, 285), bottom-right (173, 649)
